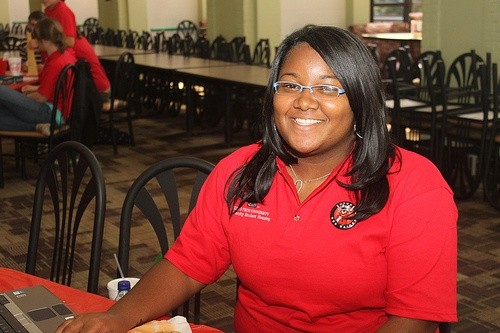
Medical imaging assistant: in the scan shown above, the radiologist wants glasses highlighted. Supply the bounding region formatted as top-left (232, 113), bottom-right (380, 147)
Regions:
top-left (273, 81), bottom-right (346, 100)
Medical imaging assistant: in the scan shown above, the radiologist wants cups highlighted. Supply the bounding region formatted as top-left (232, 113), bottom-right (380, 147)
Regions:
top-left (8, 56), bottom-right (21, 76)
top-left (106, 278), bottom-right (141, 301)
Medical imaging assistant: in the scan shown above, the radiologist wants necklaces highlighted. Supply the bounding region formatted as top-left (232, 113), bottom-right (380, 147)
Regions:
top-left (288, 164), bottom-right (332, 195)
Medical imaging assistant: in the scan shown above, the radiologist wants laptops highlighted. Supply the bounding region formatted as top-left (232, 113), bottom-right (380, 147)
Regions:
top-left (0, 285), bottom-right (78, 333)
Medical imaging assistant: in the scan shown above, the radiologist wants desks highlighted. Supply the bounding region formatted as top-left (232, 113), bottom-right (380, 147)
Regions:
top-left (92, 41), bottom-right (272, 144)
top-left (382, 99), bottom-right (499, 193)
top-left (0, 267), bottom-right (224, 333)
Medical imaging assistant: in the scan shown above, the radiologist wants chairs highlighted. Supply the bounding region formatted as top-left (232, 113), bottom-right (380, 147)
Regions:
top-left (26, 142), bottom-right (105, 293)
top-left (0, 16), bottom-right (500, 211)
top-left (110, 155), bottom-right (242, 333)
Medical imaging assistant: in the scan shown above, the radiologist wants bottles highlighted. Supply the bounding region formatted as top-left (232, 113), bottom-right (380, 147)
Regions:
top-left (113, 281), bottom-right (131, 301)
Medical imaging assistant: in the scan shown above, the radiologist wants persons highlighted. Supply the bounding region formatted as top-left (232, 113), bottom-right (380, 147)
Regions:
top-left (54, 24), bottom-right (460, 331)
top-left (0, 0), bottom-right (113, 133)
